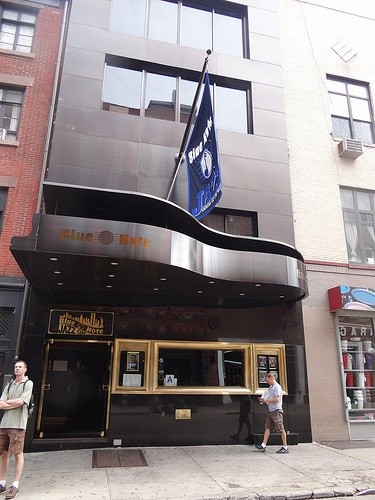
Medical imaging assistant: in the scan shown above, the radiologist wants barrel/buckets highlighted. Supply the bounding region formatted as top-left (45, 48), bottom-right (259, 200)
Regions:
top-left (353, 390), bottom-right (364, 409)
top-left (363, 341), bottom-right (372, 351)
top-left (342, 354), bottom-right (373, 387)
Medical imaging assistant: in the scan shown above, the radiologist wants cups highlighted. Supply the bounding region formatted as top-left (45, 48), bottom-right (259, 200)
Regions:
top-left (259, 397), bottom-right (263, 404)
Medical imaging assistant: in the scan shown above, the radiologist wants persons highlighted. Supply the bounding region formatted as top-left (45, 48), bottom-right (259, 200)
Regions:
top-left (254, 373), bottom-right (289, 453)
top-left (129, 355), bottom-right (137, 370)
top-left (230, 395), bottom-right (252, 441)
top-left (0, 360), bottom-right (33, 499)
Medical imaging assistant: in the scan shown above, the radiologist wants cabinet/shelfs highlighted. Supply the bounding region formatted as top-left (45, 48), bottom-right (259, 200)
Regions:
top-left (339, 322), bottom-right (375, 420)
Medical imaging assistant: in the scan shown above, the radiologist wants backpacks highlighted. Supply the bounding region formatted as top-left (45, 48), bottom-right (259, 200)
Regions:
top-left (8, 379), bottom-right (34, 417)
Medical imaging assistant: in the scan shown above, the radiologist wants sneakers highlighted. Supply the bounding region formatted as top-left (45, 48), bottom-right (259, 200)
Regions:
top-left (0, 485), bottom-right (6, 493)
top-left (6, 486), bottom-right (19, 499)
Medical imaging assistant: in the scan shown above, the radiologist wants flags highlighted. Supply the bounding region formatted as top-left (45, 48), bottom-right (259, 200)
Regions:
top-left (183, 71), bottom-right (222, 221)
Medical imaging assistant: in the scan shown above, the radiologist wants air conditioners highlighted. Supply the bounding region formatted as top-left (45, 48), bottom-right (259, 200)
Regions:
top-left (338, 138), bottom-right (364, 160)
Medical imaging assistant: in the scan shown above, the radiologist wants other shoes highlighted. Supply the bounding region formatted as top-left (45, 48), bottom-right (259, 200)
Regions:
top-left (277, 447), bottom-right (288, 453)
top-left (253, 444), bottom-right (266, 452)
